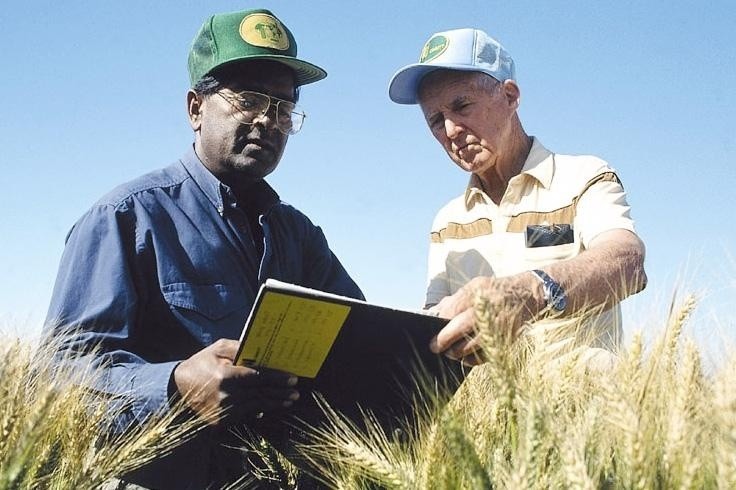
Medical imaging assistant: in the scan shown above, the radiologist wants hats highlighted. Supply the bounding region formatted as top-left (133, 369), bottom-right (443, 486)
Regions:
top-left (387, 28), bottom-right (516, 104)
top-left (187, 8), bottom-right (327, 87)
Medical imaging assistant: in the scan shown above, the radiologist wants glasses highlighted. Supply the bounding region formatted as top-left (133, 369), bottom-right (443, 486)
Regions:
top-left (216, 90), bottom-right (306, 135)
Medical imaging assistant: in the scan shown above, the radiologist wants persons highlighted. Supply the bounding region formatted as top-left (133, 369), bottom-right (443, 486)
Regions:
top-left (26, 9), bottom-right (367, 490)
top-left (389, 27), bottom-right (648, 372)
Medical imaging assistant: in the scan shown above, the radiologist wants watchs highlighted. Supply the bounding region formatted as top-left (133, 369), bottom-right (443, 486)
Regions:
top-left (532, 270), bottom-right (567, 316)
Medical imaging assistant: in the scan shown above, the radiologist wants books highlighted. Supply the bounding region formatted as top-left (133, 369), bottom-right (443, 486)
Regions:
top-left (232, 278), bottom-right (481, 443)
